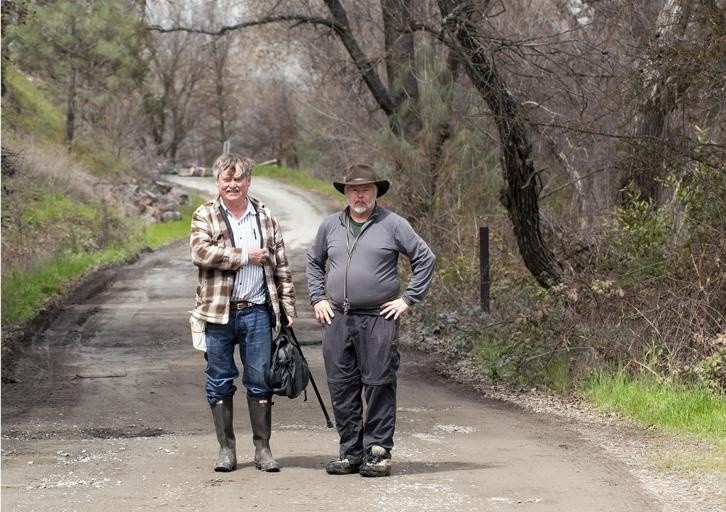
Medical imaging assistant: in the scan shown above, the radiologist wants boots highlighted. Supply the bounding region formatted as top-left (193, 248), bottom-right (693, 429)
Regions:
top-left (247, 395), bottom-right (281, 472)
top-left (208, 395), bottom-right (237, 471)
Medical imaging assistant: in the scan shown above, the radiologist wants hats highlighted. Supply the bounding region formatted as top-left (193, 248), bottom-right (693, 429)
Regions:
top-left (332, 162), bottom-right (390, 197)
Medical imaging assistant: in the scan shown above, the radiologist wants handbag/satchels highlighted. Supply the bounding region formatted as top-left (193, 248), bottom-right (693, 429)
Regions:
top-left (264, 329), bottom-right (311, 402)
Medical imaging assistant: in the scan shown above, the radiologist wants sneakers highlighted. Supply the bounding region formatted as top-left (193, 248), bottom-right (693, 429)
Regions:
top-left (325, 455), bottom-right (359, 475)
top-left (360, 444), bottom-right (392, 478)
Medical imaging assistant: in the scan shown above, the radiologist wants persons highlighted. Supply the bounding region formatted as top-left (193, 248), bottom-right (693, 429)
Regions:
top-left (188, 154), bottom-right (297, 470)
top-left (305, 163), bottom-right (437, 477)
